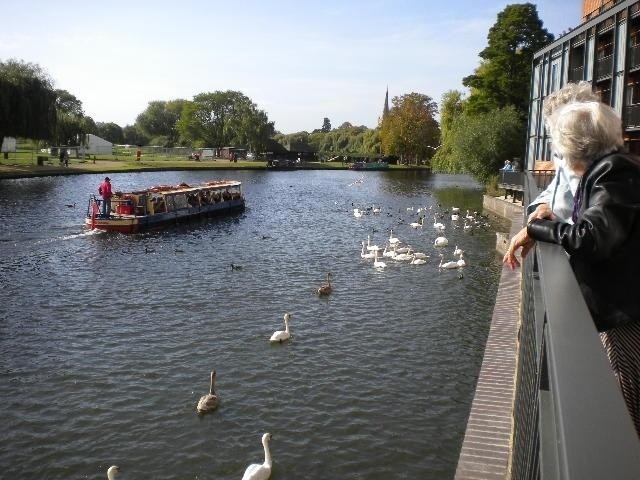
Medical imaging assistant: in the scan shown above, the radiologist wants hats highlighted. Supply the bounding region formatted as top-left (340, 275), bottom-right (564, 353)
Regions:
top-left (104, 177), bottom-right (111, 181)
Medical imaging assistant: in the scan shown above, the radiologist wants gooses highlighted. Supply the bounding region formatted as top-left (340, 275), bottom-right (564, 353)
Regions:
top-left (316, 272), bottom-right (333, 296)
top-left (240, 431), bottom-right (276, 480)
top-left (144, 248), bottom-right (156, 254)
top-left (269, 313), bottom-right (291, 344)
top-left (351, 202), bottom-right (494, 270)
top-left (64, 203), bottom-right (77, 210)
top-left (230, 263), bottom-right (243, 271)
top-left (196, 371), bottom-right (218, 416)
top-left (106, 465), bottom-right (121, 480)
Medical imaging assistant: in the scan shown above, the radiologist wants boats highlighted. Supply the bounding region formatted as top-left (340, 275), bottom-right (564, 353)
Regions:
top-left (348, 161), bottom-right (390, 171)
top-left (81, 179), bottom-right (246, 234)
top-left (266, 160), bottom-right (305, 169)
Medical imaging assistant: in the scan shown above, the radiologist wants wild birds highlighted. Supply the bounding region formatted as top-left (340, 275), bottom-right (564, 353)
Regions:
top-left (347, 176), bottom-right (364, 188)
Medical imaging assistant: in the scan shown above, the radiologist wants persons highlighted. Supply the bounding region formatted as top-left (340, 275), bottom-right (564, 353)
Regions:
top-left (229, 152), bottom-right (233, 162)
top-left (59, 152), bottom-right (65, 167)
top-left (98, 176), bottom-right (114, 216)
top-left (63, 152), bottom-right (69, 167)
top-left (233, 152), bottom-right (237, 162)
top-left (186, 187), bottom-right (241, 209)
top-left (500, 158), bottom-right (524, 202)
top-left (501, 97), bottom-right (640, 435)
top-left (525, 80), bottom-right (604, 224)
top-left (192, 152), bottom-right (200, 162)
